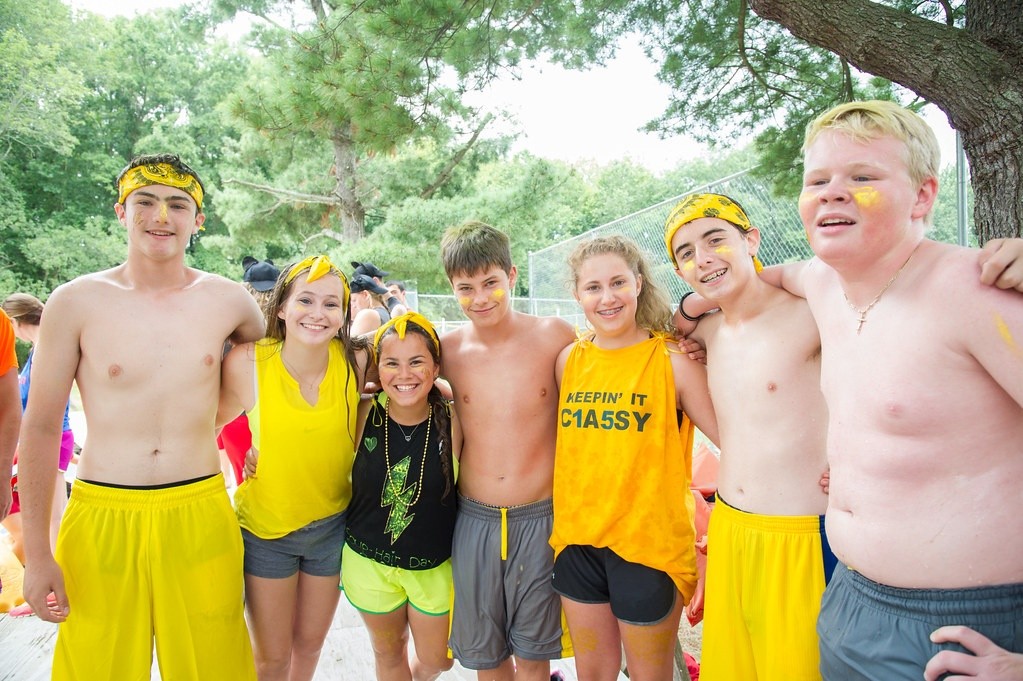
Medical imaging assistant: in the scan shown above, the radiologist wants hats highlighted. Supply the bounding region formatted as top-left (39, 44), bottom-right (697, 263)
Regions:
top-left (242, 257), bottom-right (280, 292)
top-left (351, 261), bottom-right (389, 277)
top-left (351, 276), bottom-right (386, 294)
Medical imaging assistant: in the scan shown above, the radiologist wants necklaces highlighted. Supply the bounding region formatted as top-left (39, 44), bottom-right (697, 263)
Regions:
top-left (836, 234), bottom-right (935, 332)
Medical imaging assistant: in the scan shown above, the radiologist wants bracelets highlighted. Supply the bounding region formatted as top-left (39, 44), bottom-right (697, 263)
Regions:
top-left (680, 292), bottom-right (707, 324)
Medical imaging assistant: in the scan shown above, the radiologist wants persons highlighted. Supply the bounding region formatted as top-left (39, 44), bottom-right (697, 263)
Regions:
top-left (0, 306), bottom-right (56, 616)
top-left (0, 293), bottom-right (77, 563)
top-left (668, 102), bottom-right (1019, 681)
top-left (212, 254), bottom-right (457, 681)
top-left (17, 153), bottom-right (270, 680)
top-left (428, 224), bottom-right (710, 681)
top-left (219, 250), bottom-right (286, 492)
top-left (553, 236), bottom-right (831, 681)
top-left (667, 189), bottom-right (1023, 680)
top-left (234, 311), bottom-right (461, 681)
top-left (344, 256), bottom-right (416, 393)
